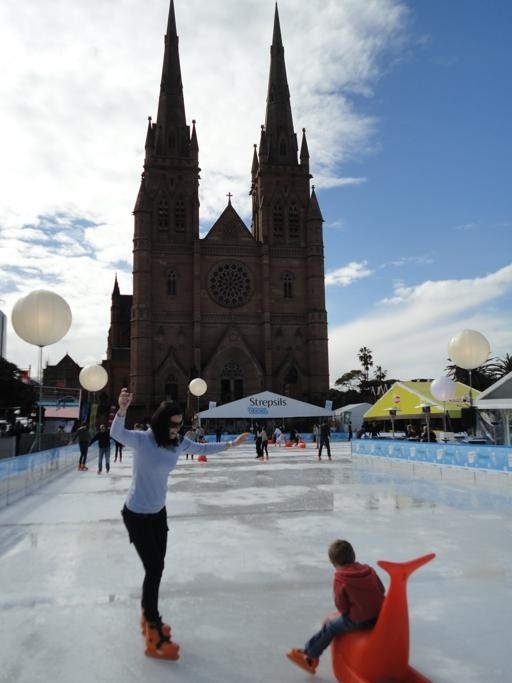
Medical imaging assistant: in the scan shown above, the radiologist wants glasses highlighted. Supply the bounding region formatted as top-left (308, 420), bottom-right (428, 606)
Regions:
top-left (170, 420), bottom-right (185, 428)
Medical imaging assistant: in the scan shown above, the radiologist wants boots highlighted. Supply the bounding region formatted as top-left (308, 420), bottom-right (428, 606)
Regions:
top-left (140, 608), bottom-right (180, 655)
top-left (78, 462), bottom-right (89, 469)
top-left (290, 645), bottom-right (319, 669)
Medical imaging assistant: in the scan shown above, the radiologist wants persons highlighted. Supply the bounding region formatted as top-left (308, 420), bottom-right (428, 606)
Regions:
top-left (109, 388), bottom-right (251, 661)
top-left (184, 425), bottom-right (205, 460)
top-left (133, 423), bottom-right (144, 430)
top-left (72, 421), bottom-right (124, 473)
top-left (406, 425), bottom-right (436, 443)
top-left (282, 539), bottom-right (386, 672)
top-left (215, 425), bottom-right (223, 442)
top-left (313, 420), bottom-right (332, 461)
top-left (253, 420), bottom-right (299, 460)
top-left (347, 421), bottom-right (353, 442)
